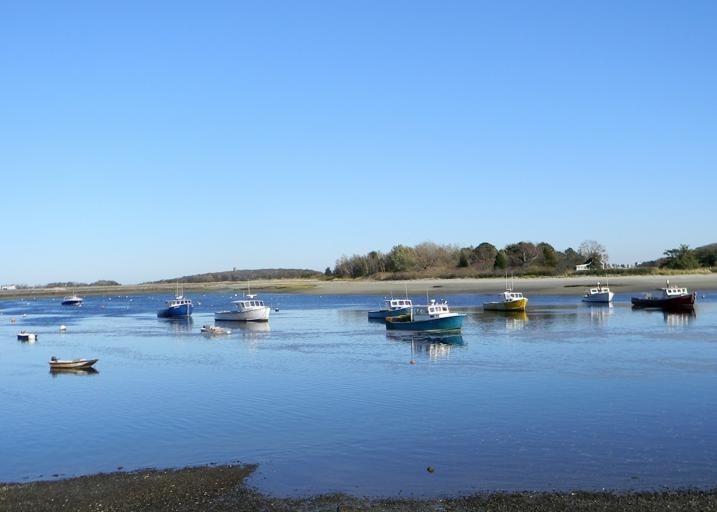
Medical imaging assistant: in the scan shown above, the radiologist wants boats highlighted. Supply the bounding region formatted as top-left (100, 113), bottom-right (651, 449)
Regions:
top-left (46, 355), bottom-right (98, 371)
top-left (366, 285), bottom-right (467, 338)
top-left (481, 271), bottom-right (530, 312)
top-left (631, 277), bottom-right (698, 312)
top-left (578, 271), bottom-right (618, 306)
top-left (60, 288), bottom-right (81, 305)
top-left (215, 277), bottom-right (273, 323)
top-left (153, 275), bottom-right (198, 320)
